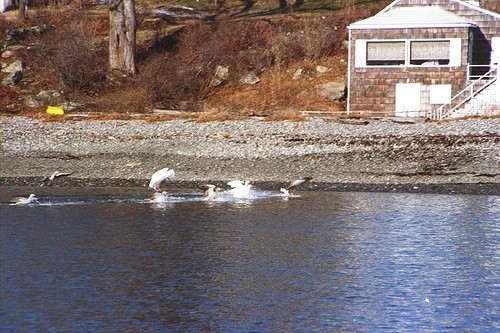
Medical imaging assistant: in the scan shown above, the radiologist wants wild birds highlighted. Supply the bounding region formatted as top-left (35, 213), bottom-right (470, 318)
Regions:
top-left (198, 177), bottom-right (314, 200)
top-left (39, 171), bottom-right (75, 187)
top-left (147, 168), bottom-right (176, 193)
top-left (11, 193), bottom-right (37, 205)
